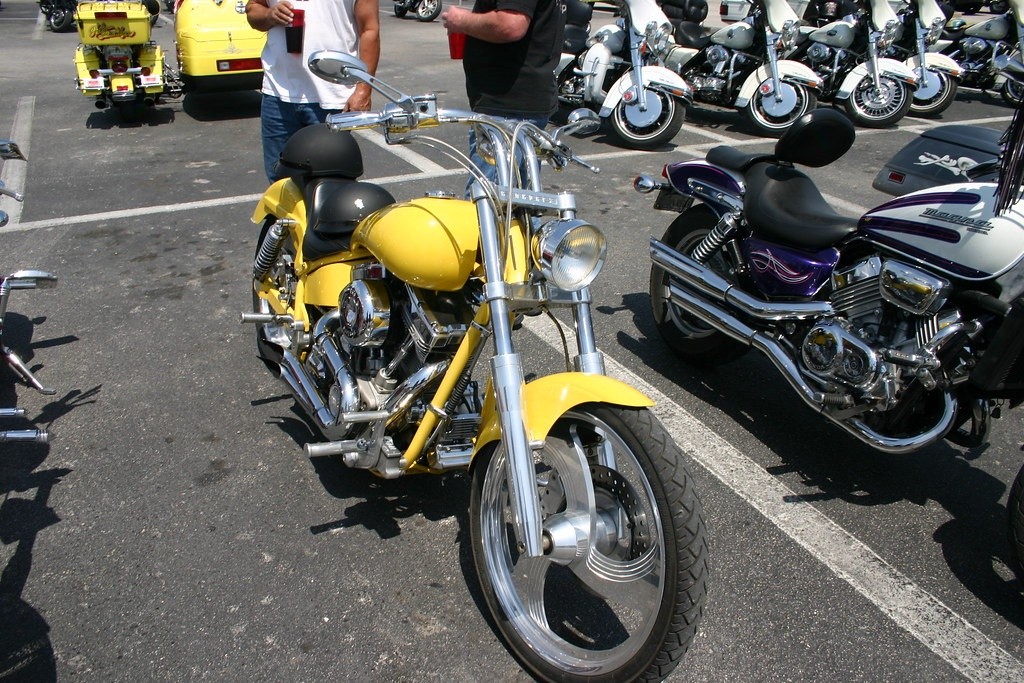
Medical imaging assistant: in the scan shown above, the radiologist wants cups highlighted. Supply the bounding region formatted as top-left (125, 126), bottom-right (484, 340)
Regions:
top-left (285, 9), bottom-right (304, 54)
top-left (448, 29), bottom-right (465, 59)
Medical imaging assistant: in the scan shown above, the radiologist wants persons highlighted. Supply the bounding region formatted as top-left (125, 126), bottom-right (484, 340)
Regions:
top-left (442, 0), bottom-right (564, 229)
top-left (243, 0), bottom-right (381, 185)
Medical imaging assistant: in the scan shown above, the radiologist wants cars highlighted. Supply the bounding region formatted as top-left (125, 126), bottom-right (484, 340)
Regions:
top-left (174, 0), bottom-right (270, 112)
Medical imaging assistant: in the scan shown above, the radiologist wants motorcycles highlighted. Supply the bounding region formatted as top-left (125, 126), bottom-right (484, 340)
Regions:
top-left (240, 49), bottom-right (713, 683)
top-left (0, 139), bottom-right (59, 495)
top-left (73, 0), bottom-right (185, 118)
top-left (551, 0), bottom-right (1024, 152)
top-left (35, 0), bottom-right (80, 32)
top-left (871, 123), bottom-right (1023, 193)
top-left (633, 106), bottom-right (1024, 575)
top-left (393, 0), bottom-right (443, 22)
